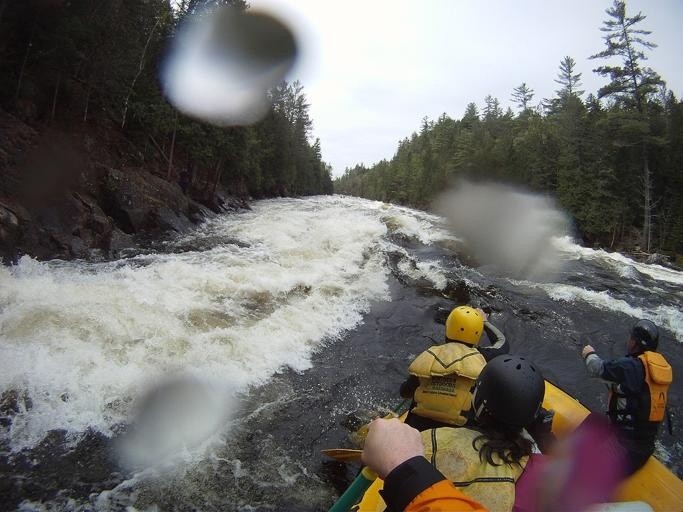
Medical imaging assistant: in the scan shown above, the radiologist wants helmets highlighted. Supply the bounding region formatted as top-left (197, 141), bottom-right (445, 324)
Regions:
top-left (445, 308), bottom-right (485, 346)
top-left (470, 351), bottom-right (545, 429)
top-left (630, 319), bottom-right (660, 352)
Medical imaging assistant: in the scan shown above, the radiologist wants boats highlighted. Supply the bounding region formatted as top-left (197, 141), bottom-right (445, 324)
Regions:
top-left (322, 356), bottom-right (682, 511)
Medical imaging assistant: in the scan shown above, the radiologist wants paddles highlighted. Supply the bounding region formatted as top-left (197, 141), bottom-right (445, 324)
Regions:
top-left (323, 448), bottom-right (360, 463)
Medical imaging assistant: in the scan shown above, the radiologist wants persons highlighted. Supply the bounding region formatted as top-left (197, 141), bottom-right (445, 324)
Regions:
top-left (382, 352), bottom-right (542, 512)
top-left (580, 318), bottom-right (673, 479)
top-left (356, 416), bottom-right (487, 512)
top-left (398, 305), bottom-right (510, 430)
